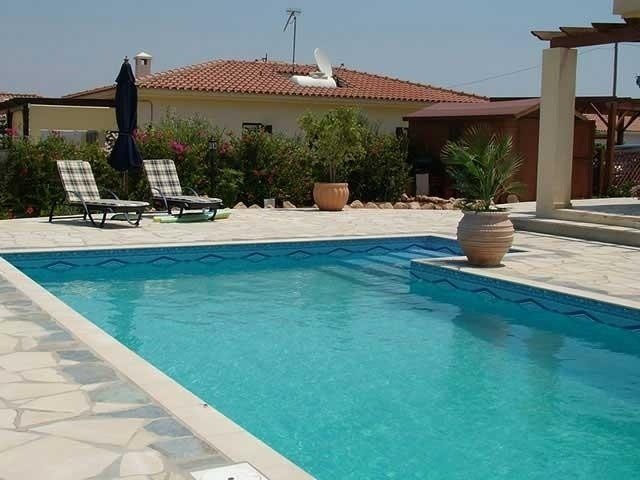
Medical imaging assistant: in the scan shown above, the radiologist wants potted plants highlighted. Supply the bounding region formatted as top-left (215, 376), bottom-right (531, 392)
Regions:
top-left (295, 107), bottom-right (367, 211)
top-left (441, 127), bottom-right (527, 266)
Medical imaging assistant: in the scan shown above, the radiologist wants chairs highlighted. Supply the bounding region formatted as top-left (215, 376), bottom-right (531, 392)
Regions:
top-left (143, 159), bottom-right (223, 221)
top-left (49, 160), bottom-right (149, 228)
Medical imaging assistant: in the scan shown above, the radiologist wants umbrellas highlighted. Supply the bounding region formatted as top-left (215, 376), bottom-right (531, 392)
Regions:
top-left (107, 56), bottom-right (143, 203)
